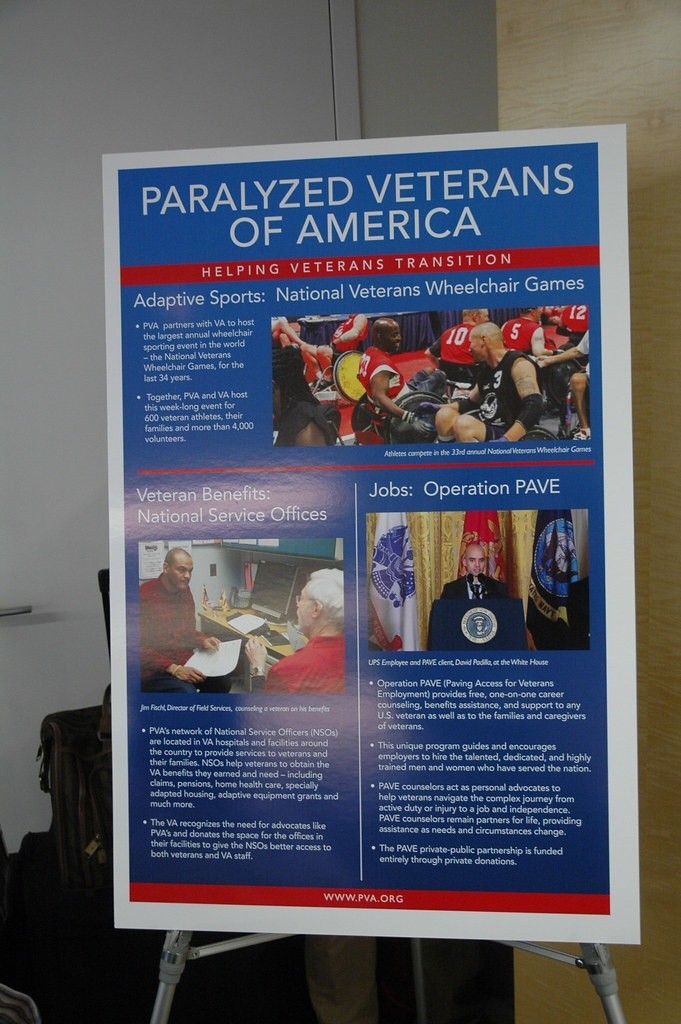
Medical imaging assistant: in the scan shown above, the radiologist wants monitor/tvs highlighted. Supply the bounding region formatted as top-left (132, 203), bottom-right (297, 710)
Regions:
top-left (251, 560), bottom-right (301, 614)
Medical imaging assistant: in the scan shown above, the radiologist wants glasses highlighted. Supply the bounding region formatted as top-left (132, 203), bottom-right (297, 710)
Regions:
top-left (295, 595), bottom-right (318, 605)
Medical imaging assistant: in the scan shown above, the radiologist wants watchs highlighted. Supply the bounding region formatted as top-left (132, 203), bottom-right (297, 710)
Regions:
top-left (250, 666), bottom-right (265, 673)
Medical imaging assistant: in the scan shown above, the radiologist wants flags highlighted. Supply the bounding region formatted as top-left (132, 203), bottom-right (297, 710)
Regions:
top-left (368, 512), bottom-right (419, 651)
top-left (456, 510), bottom-right (507, 584)
top-left (525, 509), bottom-right (587, 650)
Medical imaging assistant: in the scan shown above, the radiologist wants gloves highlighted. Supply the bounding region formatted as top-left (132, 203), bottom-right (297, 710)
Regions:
top-left (410, 401), bottom-right (440, 417)
top-left (401, 410), bottom-right (434, 435)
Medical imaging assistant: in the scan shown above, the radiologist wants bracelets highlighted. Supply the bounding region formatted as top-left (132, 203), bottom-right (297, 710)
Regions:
top-left (173, 665), bottom-right (181, 676)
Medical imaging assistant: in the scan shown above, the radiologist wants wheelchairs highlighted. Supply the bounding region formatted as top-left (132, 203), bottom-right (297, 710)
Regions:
top-left (541, 347), bottom-right (583, 411)
top-left (437, 359), bottom-right (500, 421)
top-left (349, 384), bottom-right (448, 444)
top-left (519, 419), bottom-right (561, 442)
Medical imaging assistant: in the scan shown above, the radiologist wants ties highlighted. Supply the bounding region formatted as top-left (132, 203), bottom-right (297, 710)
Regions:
top-left (470, 584), bottom-right (482, 600)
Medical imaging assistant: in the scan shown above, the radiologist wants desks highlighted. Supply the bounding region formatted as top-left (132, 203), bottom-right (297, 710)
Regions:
top-left (197, 604), bottom-right (309, 692)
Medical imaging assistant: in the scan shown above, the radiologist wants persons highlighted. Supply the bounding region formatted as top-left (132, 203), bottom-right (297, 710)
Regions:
top-left (423, 305), bottom-right (591, 440)
top-left (439, 543), bottom-right (509, 599)
top-left (272, 314), bottom-right (370, 447)
top-left (418, 322), bottom-right (542, 443)
top-left (245, 568), bottom-right (344, 697)
top-left (350, 317), bottom-right (446, 444)
top-left (140, 548), bottom-right (232, 695)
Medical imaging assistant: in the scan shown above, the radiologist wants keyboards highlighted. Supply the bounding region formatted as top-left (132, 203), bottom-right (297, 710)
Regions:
top-left (227, 612), bottom-right (270, 636)
top-left (261, 630), bottom-right (290, 647)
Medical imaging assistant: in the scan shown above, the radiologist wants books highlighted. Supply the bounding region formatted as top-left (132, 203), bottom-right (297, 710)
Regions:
top-left (261, 630), bottom-right (290, 646)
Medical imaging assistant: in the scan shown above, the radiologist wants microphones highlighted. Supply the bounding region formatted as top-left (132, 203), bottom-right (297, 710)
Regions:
top-left (467, 574), bottom-right (474, 591)
top-left (477, 573), bottom-right (489, 587)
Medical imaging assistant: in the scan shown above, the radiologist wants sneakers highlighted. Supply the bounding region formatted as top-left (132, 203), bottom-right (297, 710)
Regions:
top-left (573, 427), bottom-right (592, 442)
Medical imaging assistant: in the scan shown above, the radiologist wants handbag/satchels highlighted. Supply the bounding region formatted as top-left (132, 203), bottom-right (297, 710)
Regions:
top-left (35, 704), bottom-right (111, 901)
